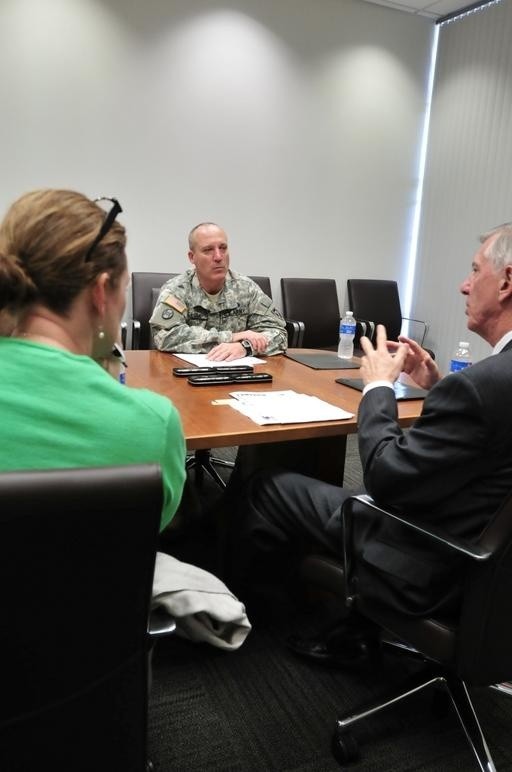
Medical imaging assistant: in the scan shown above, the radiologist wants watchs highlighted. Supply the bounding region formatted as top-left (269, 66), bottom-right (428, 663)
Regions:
top-left (239, 340), bottom-right (252, 357)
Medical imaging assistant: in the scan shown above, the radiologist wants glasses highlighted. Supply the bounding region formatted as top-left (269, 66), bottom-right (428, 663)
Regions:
top-left (85, 197), bottom-right (123, 265)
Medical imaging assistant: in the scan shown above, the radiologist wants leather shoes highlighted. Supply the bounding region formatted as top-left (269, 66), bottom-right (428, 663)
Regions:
top-left (286, 620), bottom-right (382, 668)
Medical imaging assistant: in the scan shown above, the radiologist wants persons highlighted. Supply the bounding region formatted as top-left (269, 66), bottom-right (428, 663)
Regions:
top-left (226, 222), bottom-right (512, 660)
top-left (0, 188), bottom-right (187, 535)
top-left (149, 223), bottom-right (288, 363)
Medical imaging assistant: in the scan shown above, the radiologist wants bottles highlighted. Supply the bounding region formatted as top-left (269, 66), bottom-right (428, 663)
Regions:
top-left (448, 341), bottom-right (471, 376)
top-left (337, 311), bottom-right (356, 359)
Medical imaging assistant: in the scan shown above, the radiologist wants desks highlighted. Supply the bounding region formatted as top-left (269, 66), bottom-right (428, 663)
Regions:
top-left (105, 350), bottom-right (433, 487)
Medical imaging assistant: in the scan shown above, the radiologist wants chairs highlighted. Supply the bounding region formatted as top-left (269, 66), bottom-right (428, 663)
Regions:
top-left (287, 481), bottom-right (512, 772)
top-left (281, 276), bottom-right (344, 350)
top-left (129, 267), bottom-right (240, 494)
top-left (244, 276), bottom-right (299, 347)
top-left (0, 466), bottom-right (181, 771)
top-left (345, 274), bottom-right (436, 362)
